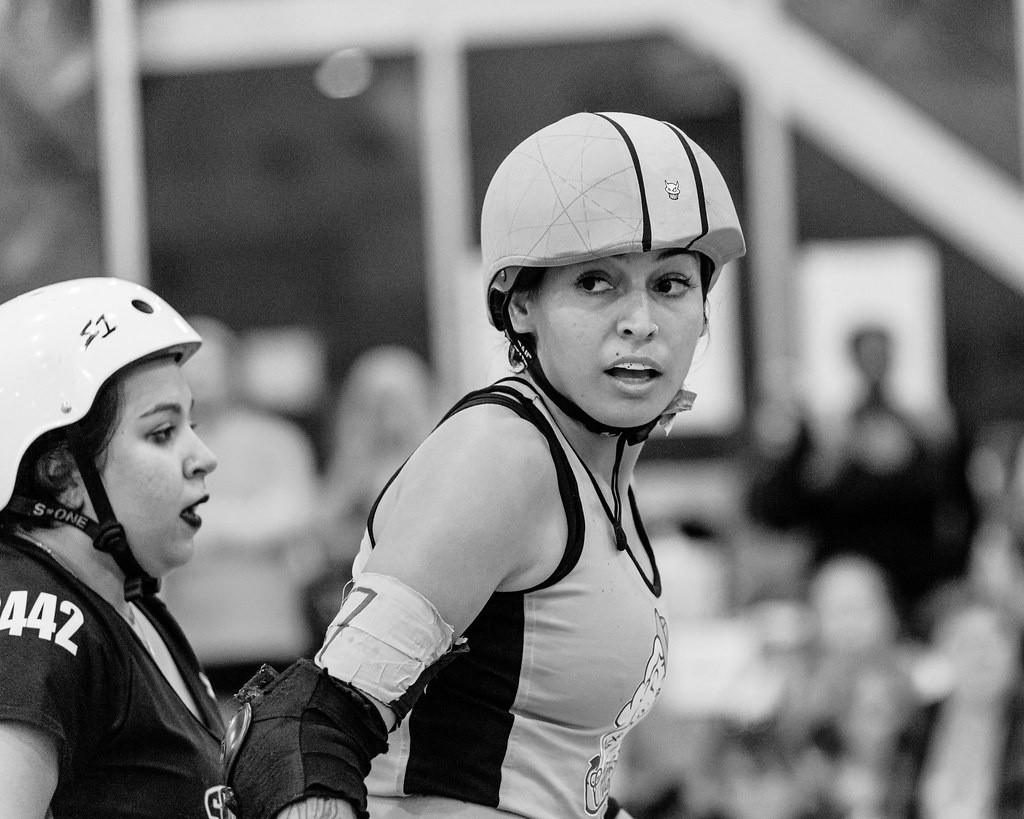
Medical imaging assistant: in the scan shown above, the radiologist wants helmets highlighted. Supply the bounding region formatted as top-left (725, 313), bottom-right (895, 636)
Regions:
top-left (0, 275), bottom-right (205, 520)
top-left (480, 111), bottom-right (748, 331)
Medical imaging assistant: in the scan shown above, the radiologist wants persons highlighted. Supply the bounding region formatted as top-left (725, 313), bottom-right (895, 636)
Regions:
top-left (269, 110), bottom-right (749, 819)
top-left (0, 276), bottom-right (228, 817)
